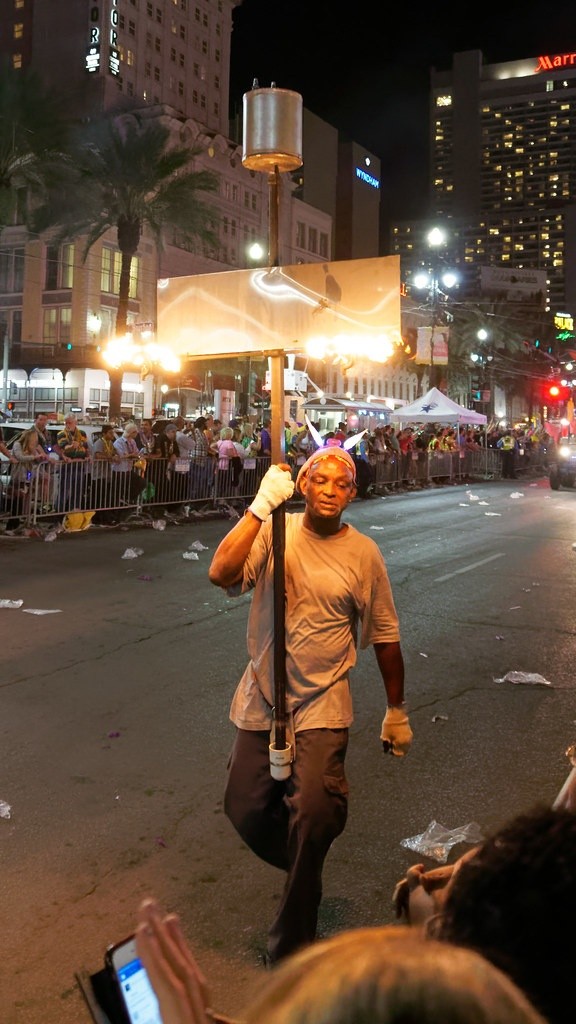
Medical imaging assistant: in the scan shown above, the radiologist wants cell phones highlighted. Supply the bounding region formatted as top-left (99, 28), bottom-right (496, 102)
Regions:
top-left (104, 934), bottom-right (163, 1024)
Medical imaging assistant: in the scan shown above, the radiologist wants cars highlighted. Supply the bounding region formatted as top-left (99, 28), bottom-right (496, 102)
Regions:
top-left (549, 441), bottom-right (576, 491)
top-left (0, 423), bottom-right (124, 509)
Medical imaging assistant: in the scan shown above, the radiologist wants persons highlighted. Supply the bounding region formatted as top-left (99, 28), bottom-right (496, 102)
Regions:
top-left (0, 409), bottom-right (576, 538)
top-left (136, 803), bottom-right (575, 1024)
top-left (209, 445), bottom-right (413, 970)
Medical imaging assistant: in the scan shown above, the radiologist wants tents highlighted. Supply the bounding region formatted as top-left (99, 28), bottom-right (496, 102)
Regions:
top-left (392, 387), bottom-right (489, 472)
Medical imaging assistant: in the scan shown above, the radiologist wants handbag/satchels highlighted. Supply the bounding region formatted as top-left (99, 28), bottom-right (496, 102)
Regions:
top-left (243, 458), bottom-right (256, 469)
top-left (496, 438), bottom-right (504, 449)
top-left (62, 511), bottom-right (96, 532)
top-left (174, 464), bottom-right (189, 471)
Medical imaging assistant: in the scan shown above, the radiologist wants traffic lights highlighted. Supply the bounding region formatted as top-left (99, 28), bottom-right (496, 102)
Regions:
top-left (8, 403), bottom-right (15, 410)
top-left (61, 342), bottom-right (73, 351)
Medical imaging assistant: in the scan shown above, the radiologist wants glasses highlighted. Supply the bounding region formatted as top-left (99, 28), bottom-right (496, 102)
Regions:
top-left (420, 914), bottom-right (445, 940)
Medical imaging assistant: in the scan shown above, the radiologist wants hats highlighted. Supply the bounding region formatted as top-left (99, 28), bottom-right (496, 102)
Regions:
top-left (296, 414), bottom-right (368, 493)
top-left (165, 424), bottom-right (178, 433)
top-left (337, 422), bottom-right (347, 430)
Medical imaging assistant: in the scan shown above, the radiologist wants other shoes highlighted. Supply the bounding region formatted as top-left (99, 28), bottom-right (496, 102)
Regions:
top-left (265, 951), bottom-right (273, 971)
